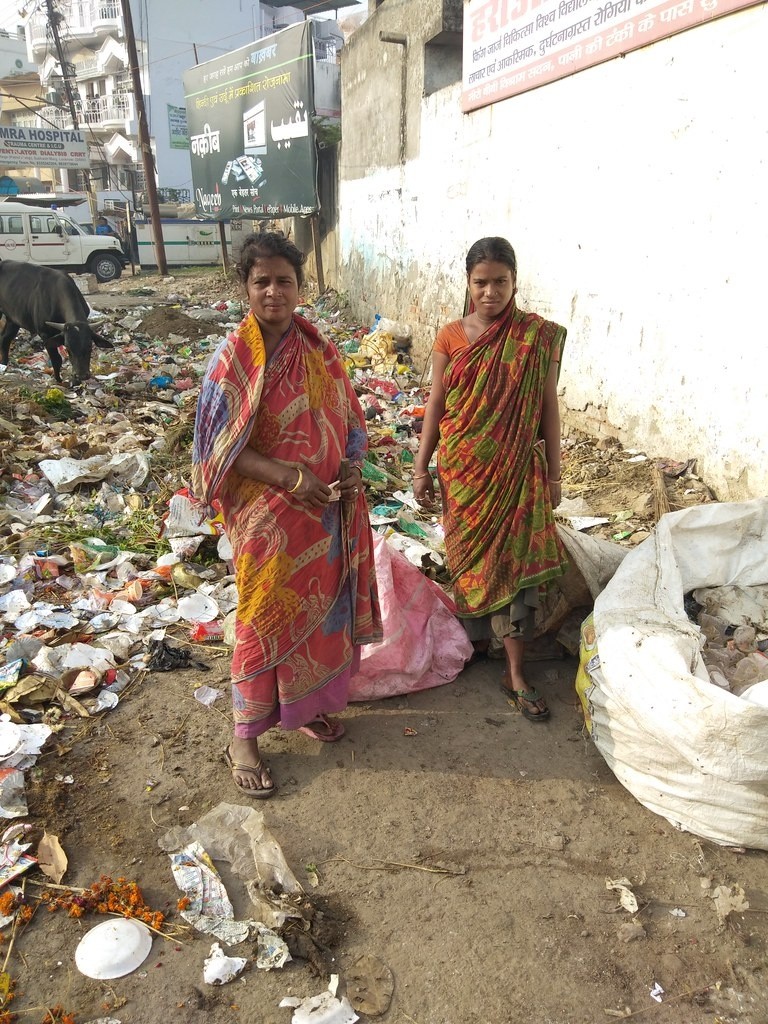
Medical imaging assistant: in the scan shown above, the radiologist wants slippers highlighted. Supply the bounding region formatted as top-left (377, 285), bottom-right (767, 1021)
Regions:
top-left (223, 745), bottom-right (276, 799)
top-left (277, 714), bottom-right (345, 742)
top-left (464, 650), bottom-right (488, 667)
top-left (500, 684), bottom-right (550, 721)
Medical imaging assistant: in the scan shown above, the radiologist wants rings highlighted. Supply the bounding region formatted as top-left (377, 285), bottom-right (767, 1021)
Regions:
top-left (413, 497), bottom-right (426, 501)
top-left (560, 499), bottom-right (562, 502)
top-left (353, 486), bottom-right (359, 496)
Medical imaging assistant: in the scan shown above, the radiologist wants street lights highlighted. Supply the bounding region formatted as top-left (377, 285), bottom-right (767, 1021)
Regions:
top-left (124, 168), bottom-right (137, 212)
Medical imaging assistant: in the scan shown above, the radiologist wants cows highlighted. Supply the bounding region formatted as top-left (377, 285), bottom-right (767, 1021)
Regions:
top-left (0, 258), bottom-right (115, 385)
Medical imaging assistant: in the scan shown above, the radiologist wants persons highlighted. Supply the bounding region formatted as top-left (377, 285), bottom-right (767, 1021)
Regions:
top-left (213, 233), bottom-right (369, 790)
top-left (413, 235), bottom-right (562, 718)
top-left (97, 217), bottom-right (115, 235)
top-left (124, 218), bottom-right (138, 264)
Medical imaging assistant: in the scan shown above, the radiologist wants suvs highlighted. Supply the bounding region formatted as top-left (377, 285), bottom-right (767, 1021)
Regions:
top-left (0, 200), bottom-right (127, 283)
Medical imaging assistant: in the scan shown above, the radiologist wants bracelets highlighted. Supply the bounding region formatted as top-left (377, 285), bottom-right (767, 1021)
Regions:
top-left (547, 477), bottom-right (562, 484)
top-left (287, 469), bottom-right (303, 493)
top-left (352, 466), bottom-right (363, 479)
top-left (413, 472), bottom-right (429, 479)
